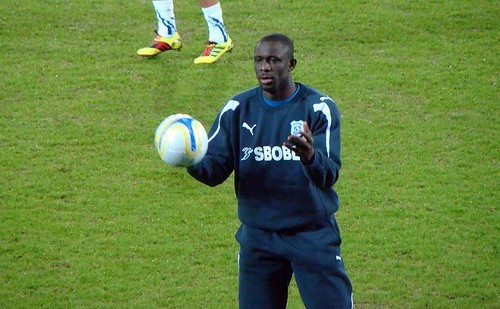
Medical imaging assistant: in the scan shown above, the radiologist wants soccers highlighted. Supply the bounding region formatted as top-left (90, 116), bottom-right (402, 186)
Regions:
top-left (154, 114), bottom-right (210, 168)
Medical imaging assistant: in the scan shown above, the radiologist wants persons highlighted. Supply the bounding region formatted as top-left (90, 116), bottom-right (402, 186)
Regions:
top-left (184, 32), bottom-right (355, 309)
top-left (136, 0), bottom-right (234, 65)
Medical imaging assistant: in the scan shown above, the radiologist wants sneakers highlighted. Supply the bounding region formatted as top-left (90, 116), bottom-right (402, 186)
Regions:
top-left (195, 34), bottom-right (234, 63)
top-left (137, 31), bottom-right (183, 56)
